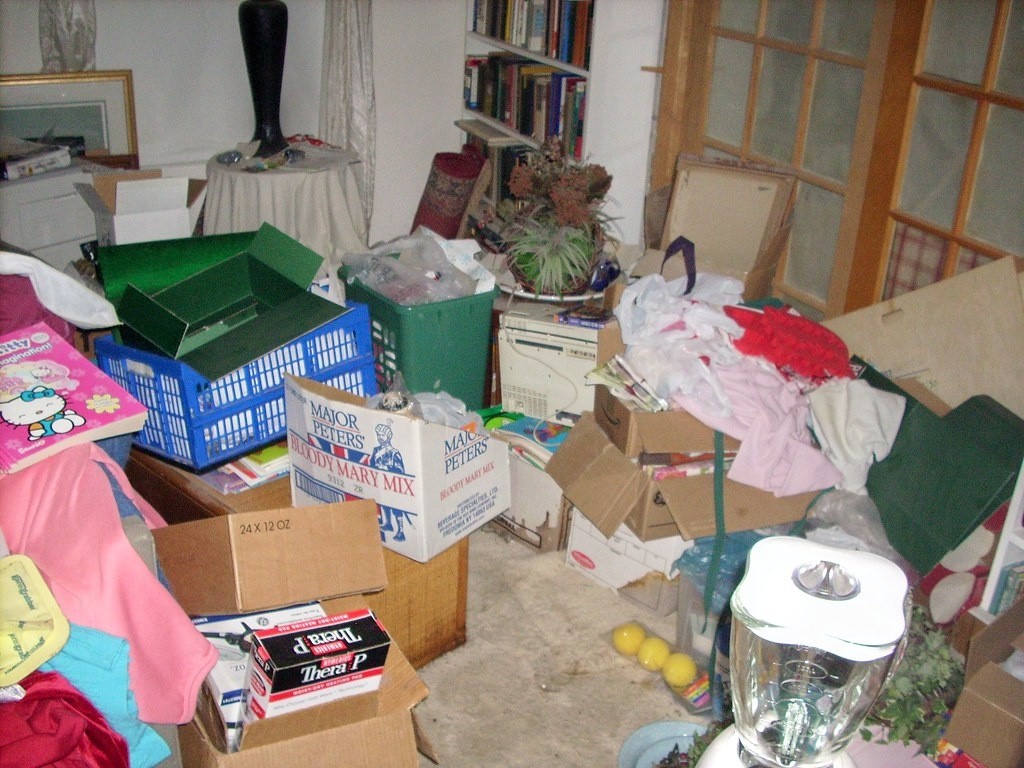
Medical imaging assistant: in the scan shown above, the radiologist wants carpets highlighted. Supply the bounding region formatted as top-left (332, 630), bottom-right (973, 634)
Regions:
top-left (410, 144), bottom-right (486, 239)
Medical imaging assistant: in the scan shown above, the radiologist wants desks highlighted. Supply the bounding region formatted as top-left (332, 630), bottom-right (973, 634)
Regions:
top-left (202, 140), bottom-right (370, 276)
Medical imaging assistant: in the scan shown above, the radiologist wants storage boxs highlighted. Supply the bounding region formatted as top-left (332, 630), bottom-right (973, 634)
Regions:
top-left (72, 168), bottom-right (1024, 768)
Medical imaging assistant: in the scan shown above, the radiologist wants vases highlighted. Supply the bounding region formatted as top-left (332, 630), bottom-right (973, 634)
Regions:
top-left (507, 199), bottom-right (606, 296)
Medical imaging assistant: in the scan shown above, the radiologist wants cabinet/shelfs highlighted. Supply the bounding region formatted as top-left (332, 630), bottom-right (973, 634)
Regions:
top-left (453, 0), bottom-right (667, 246)
top-left (0, 157), bottom-right (118, 269)
top-left (969, 462), bottom-right (1024, 626)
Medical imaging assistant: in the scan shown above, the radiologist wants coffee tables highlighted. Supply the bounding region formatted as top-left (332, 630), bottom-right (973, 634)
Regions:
top-left (123, 438), bottom-right (470, 669)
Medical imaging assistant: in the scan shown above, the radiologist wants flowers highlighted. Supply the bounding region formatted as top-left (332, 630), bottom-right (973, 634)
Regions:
top-left (488, 134), bottom-right (627, 304)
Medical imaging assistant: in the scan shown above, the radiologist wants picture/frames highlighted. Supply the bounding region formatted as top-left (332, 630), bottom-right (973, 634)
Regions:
top-left (0, 69), bottom-right (140, 171)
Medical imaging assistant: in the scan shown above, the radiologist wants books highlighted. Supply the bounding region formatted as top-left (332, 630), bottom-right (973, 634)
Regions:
top-left (0, 321), bottom-right (148, 473)
top-left (462, 0), bottom-right (596, 225)
top-left (582, 353), bottom-right (669, 411)
top-left (553, 305), bottom-right (618, 328)
top-left (989, 561), bottom-right (1024, 616)
top-left (496, 417), bottom-right (573, 466)
top-left (200, 442), bottom-right (290, 496)
